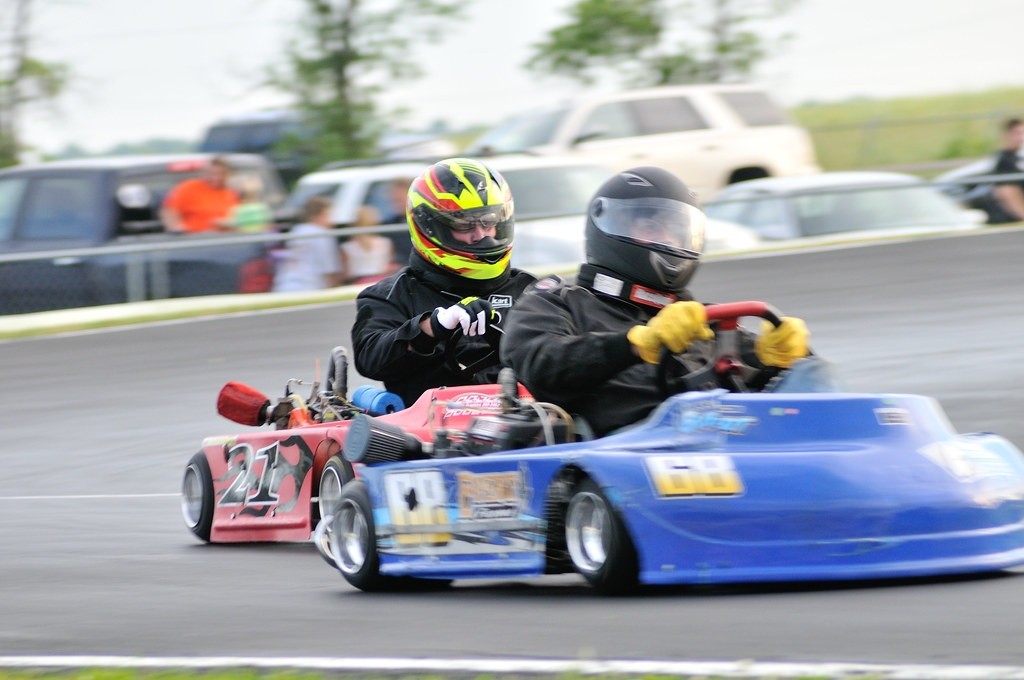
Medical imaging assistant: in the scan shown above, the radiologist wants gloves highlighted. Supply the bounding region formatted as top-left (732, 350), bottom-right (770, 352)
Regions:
top-left (753, 317), bottom-right (811, 369)
top-left (430, 297), bottom-right (496, 341)
top-left (626, 301), bottom-right (715, 364)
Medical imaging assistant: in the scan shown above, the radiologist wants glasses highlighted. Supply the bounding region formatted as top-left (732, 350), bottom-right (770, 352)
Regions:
top-left (450, 211), bottom-right (498, 230)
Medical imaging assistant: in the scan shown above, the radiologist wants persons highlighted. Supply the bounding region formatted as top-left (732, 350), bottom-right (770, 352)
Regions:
top-left (157, 154), bottom-right (274, 236)
top-left (265, 179), bottom-right (435, 294)
top-left (499, 163), bottom-right (813, 451)
top-left (989, 117), bottom-right (1024, 223)
top-left (351, 157), bottom-right (537, 403)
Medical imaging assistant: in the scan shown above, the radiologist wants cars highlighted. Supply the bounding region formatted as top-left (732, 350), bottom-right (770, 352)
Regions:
top-left (2, 72), bottom-right (1019, 324)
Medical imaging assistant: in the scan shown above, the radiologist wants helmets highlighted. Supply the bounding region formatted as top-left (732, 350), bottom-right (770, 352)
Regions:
top-left (585, 166), bottom-right (707, 293)
top-left (404, 157), bottom-right (514, 281)
